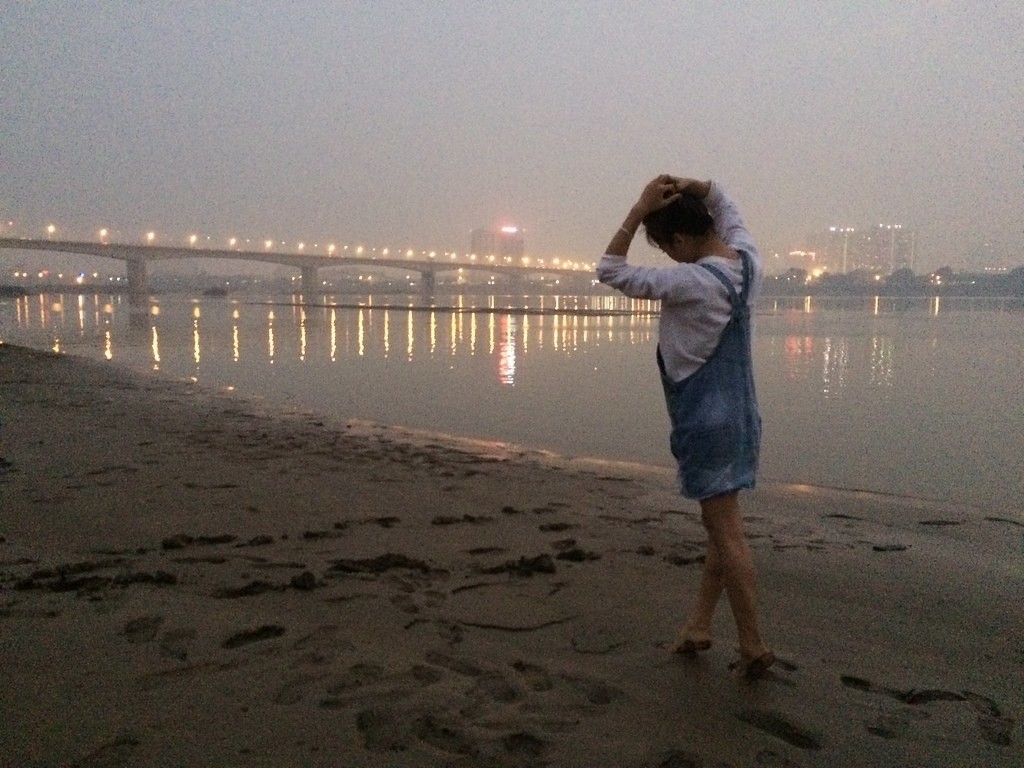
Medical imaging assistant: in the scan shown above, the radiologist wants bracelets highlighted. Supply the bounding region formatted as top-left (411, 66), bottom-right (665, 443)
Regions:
top-left (619, 226), bottom-right (634, 238)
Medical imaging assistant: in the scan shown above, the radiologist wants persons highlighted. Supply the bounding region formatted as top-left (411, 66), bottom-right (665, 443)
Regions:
top-left (597, 174), bottom-right (775, 678)
